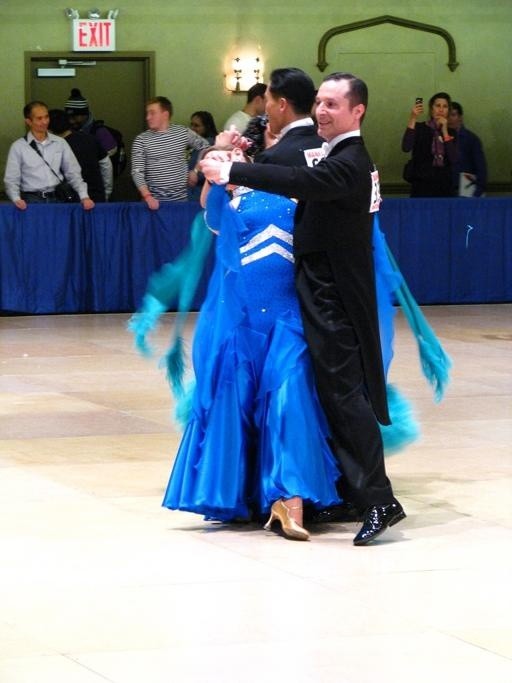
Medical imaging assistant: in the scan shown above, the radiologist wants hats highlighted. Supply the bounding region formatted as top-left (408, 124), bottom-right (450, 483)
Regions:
top-left (64, 88), bottom-right (88, 110)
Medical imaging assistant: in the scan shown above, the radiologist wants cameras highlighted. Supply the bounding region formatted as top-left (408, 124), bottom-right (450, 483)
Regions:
top-left (415, 98), bottom-right (423, 107)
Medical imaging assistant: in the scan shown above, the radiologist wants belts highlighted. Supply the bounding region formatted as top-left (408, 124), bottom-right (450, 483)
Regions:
top-left (32, 191), bottom-right (55, 200)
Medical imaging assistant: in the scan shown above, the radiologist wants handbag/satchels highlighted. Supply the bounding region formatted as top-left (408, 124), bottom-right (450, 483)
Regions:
top-left (54, 181), bottom-right (78, 203)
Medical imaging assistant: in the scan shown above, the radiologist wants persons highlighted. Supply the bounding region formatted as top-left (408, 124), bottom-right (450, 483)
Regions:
top-left (401, 90), bottom-right (461, 198)
top-left (62, 87), bottom-right (121, 202)
top-left (47, 107), bottom-right (114, 201)
top-left (446, 101), bottom-right (488, 196)
top-left (185, 110), bottom-right (217, 202)
top-left (198, 65), bottom-right (406, 548)
top-left (130, 95), bottom-right (209, 211)
top-left (3, 99), bottom-right (96, 211)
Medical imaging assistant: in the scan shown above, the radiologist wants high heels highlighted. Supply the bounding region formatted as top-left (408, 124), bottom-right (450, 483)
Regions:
top-left (263, 499), bottom-right (309, 541)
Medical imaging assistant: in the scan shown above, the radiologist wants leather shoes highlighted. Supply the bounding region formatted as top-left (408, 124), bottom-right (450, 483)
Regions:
top-left (352, 498), bottom-right (407, 546)
top-left (316, 500), bottom-right (360, 522)
